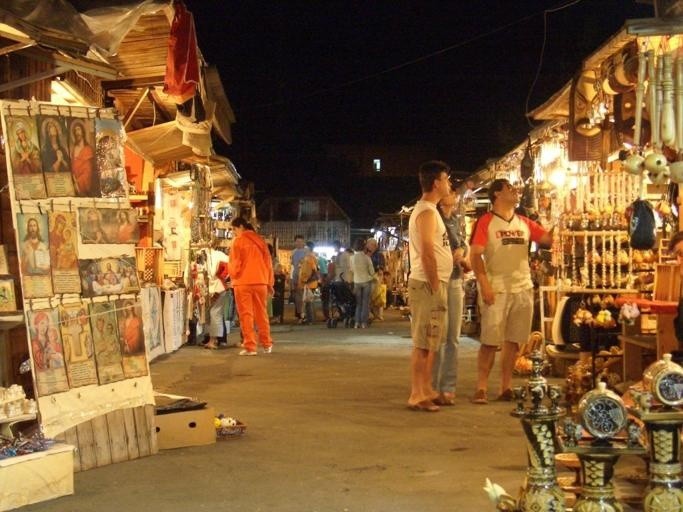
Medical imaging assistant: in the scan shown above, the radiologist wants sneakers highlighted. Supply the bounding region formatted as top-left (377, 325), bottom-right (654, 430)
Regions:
top-left (238, 349), bottom-right (258, 356)
top-left (262, 344), bottom-right (273, 354)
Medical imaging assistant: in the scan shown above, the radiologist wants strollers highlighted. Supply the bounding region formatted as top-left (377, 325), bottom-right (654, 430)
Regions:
top-left (322, 272), bottom-right (357, 329)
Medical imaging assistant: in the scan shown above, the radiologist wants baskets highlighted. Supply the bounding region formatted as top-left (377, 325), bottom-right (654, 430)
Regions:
top-left (215, 419), bottom-right (247, 441)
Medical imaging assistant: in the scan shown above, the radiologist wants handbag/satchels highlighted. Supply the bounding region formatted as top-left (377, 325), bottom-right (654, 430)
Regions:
top-left (370, 282), bottom-right (387, 307)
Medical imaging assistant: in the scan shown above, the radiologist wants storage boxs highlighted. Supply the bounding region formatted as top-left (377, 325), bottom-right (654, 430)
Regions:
top-left (0, 441), bottom-right (78, 512)
top-left (154, 393), bottom-right (218, 454)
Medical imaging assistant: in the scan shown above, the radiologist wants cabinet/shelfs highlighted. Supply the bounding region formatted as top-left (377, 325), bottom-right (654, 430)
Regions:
top-left (614, 294), bottom-right (683, 398)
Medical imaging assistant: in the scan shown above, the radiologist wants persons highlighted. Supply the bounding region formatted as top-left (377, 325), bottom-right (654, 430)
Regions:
top-left (71, 124), bottom-right (91, 183)
top-left (409, 160), bottom-right (456, 414)
top-left (82, 262), bottom-right (137, 291)
top-left (81, 210), bottom-right (105, 243)
top-left (123, 302), bottom-right (145, 354)
top-left (40, 118), bottom-right (67, 172)
top-left (183, 245), bottom-right (235, 351)
top-left (229, 218), bottom-right (277, 359)
top-left (115, 209), bottom-right (133, 244)
top-left (433, 181), bottom-right (465, 405)
top-left (470, 178), bottom-right (559, 405)
top-left (30, 315), bottom-right (61, 366)
top-left (290, 229), bottom-right (389, 329)
top-left (93, 311), bottom-right (116, 361)
top-left (19, 217), bottom-right (47, 273)
top-left (9, 121), bottom-right (32, 169)
top-left (50, 216), bottom-right (75, 266)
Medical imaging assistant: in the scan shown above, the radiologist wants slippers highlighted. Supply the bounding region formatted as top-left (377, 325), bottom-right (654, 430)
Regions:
top-left (498, 388), bottom-right (516, 402)
top-left (406, 398), bottom-right (440, 412)
top-left (431, 392), bottom-right (455, 406)
top-left (472, 389), bottom-right (489, 404)
